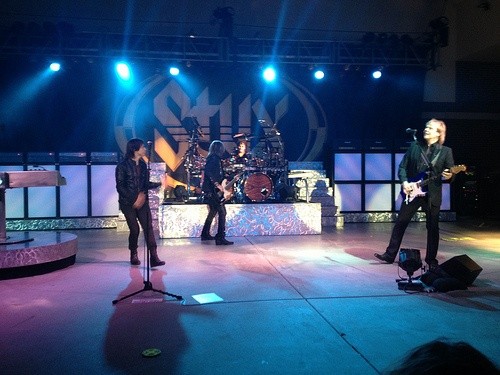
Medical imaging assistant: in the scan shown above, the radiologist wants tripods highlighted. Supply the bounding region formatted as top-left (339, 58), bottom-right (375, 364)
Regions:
top-left (112, 150), bottom-right (182, 305)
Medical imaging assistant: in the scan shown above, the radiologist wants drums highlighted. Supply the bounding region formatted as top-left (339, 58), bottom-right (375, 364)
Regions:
top-left (224, 152), bottom-right (274, 204)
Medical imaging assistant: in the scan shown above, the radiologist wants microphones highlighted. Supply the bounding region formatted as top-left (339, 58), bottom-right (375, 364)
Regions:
top-left (405, 128), bottom-right (417, 133)
top-left (147, 141), bottom-right (152, 150)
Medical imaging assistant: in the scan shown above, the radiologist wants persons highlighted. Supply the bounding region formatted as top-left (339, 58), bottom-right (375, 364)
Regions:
top-left (374, 119), bottom-right (454, 265)
top-left (383, 339), bottom-right (500, 375)
top-left (232, 140), bottom-right (253, 173)
top-left (200, 140), bottom-right (240, 246)
top-left (116, 138), bottom-right (165, 267)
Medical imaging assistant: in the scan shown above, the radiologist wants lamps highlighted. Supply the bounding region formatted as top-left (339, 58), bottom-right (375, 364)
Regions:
top-left (398, 246), bottom-right (425, 279)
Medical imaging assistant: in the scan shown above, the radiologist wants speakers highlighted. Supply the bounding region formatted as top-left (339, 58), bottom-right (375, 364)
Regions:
top-left (420, 254), bottom-right (483, 293)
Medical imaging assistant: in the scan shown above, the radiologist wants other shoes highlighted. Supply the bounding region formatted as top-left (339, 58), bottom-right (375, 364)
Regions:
top-left (150, 256), bottom-right (165, 268)
top-left (214, 235), bottom-right (234, 246)
top-left (131, 251), bottom-right (140, 265)
top-left (201, 234), bottom-right (215, 240)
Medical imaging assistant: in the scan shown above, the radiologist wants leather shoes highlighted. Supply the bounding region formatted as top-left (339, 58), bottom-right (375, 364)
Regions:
top-left (428, 262), bottom-right (438, 269)
top-left (375, 253), bottom-right (395, 263)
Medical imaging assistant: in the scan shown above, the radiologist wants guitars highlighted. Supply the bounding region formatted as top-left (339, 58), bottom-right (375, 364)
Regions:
top-left (403, 163), bottom-right (469, 205)
top-left (212, 170), bottom-right (245, 203)
